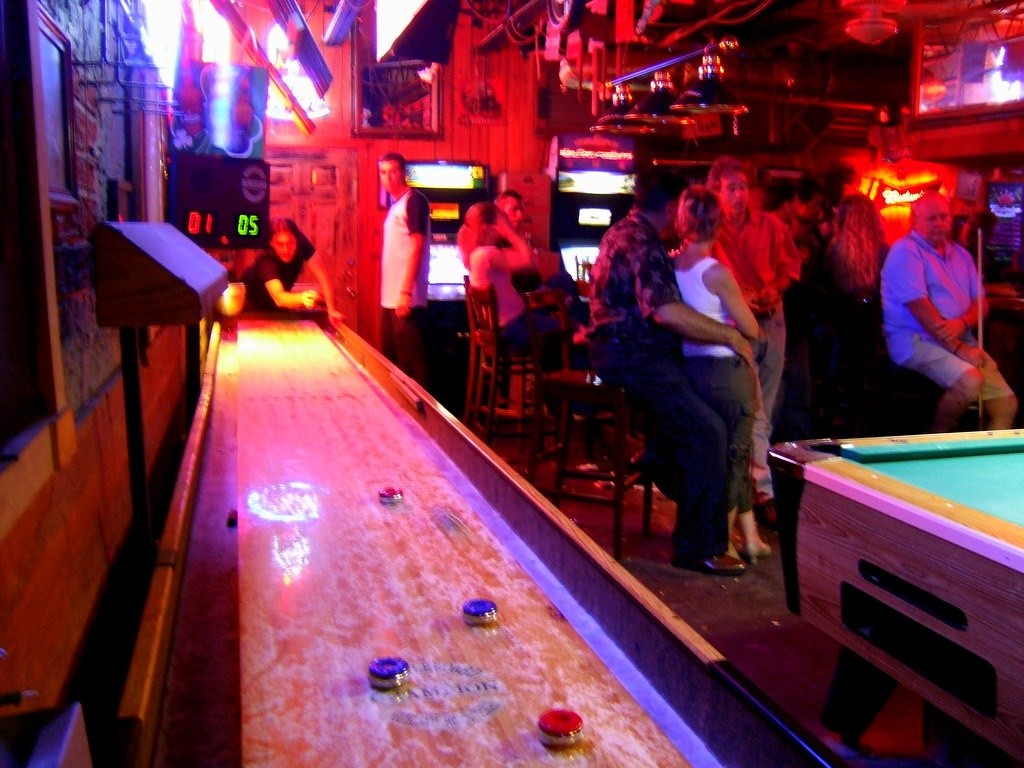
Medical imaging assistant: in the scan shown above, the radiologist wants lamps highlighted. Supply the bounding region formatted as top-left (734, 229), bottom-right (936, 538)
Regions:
top-left (623, 70), bottom-right (697, 125)
top-left (669, 56), bottom-right (749, 113)
top-left (590, 84), bottom-right (656, 135)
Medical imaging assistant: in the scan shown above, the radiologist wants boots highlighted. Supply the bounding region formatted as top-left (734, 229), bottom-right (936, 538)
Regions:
top-left (728, 505), bottom-right (771, 564)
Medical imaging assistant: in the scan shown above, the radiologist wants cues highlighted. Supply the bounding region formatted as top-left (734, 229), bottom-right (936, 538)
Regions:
top-left (977, 227), bottom-right (984, 431)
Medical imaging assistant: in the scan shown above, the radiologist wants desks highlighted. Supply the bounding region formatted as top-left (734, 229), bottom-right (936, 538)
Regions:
top-left (766, 429), bottom-right (1024, 768)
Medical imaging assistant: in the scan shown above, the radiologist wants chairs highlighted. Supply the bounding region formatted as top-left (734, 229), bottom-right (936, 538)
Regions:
top-left (853, 288), bottom-right (939, 436)
top-left (463, 274), bottom-right (653, 563)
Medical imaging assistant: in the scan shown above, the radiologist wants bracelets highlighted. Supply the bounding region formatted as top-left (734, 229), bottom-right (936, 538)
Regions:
top-left (400, 290), bottom-right (413, 297)
top-left (960, 316), bottom-right (969, 332)
top-left (953, 342), bottom-right (962, 353)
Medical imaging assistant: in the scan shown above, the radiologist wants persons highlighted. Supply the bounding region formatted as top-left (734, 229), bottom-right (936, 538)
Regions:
top-left (459, 153), bottom-right (891, 520)
top-left (378, 152), bottom-right (430, 384)
top-left (245, 217), bottom-right (341, 320)
top-left (671, 190), bottom-right (772, 567)
top-left (880, 191), bottom-right (1018, 434)
top-left (592, 163), bottom-right (747, 575)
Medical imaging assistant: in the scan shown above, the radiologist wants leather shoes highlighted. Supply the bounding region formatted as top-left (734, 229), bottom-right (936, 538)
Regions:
top-left (673, 548), bottom-right (746, 576)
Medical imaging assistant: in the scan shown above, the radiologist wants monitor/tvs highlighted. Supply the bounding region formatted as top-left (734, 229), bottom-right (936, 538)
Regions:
top-left (560, 247), bottom-right (601, 283)
top-left (428, 243), bottom-right (471, 284)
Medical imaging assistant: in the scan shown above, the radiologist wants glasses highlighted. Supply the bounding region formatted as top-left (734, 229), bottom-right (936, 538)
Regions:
top-left (513, 204), bottom-right (530, 213)
